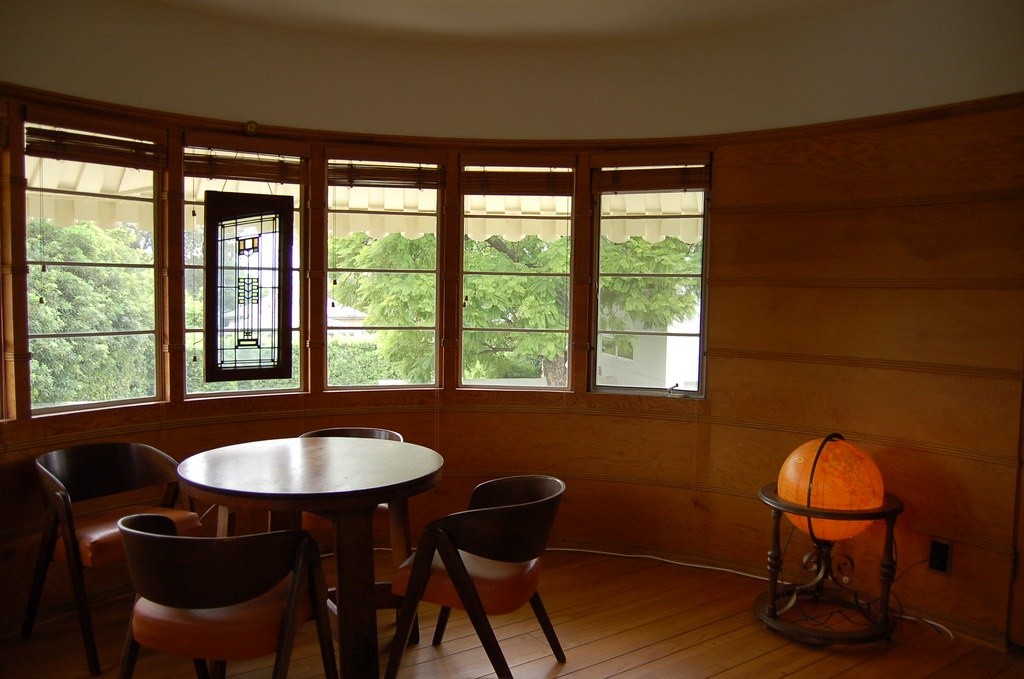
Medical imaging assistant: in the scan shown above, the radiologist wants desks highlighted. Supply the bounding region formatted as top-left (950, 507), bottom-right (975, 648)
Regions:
top-left (176, 437), bottom-right (445, 679)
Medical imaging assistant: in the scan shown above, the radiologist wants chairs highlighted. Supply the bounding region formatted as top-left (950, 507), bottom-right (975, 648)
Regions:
top-left (384, 474), bottom-right (567, 679)
top-left (116, 512), bottom-right (340, 679)
top-left (19, 441), bottom-right (203, 679)
top-left (269, 426), bottom-right (404, 519)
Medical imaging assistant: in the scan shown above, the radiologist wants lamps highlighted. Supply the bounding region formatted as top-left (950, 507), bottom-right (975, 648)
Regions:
top-left (753, 432), bottom-right (906, 650)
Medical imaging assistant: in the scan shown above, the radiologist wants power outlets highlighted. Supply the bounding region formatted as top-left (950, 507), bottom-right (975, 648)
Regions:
top-left (927, 539), bottom-right (955, 577)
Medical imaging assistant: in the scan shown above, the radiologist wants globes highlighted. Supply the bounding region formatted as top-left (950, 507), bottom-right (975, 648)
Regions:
top-left (752, 434), bottom-right (904, 642)
